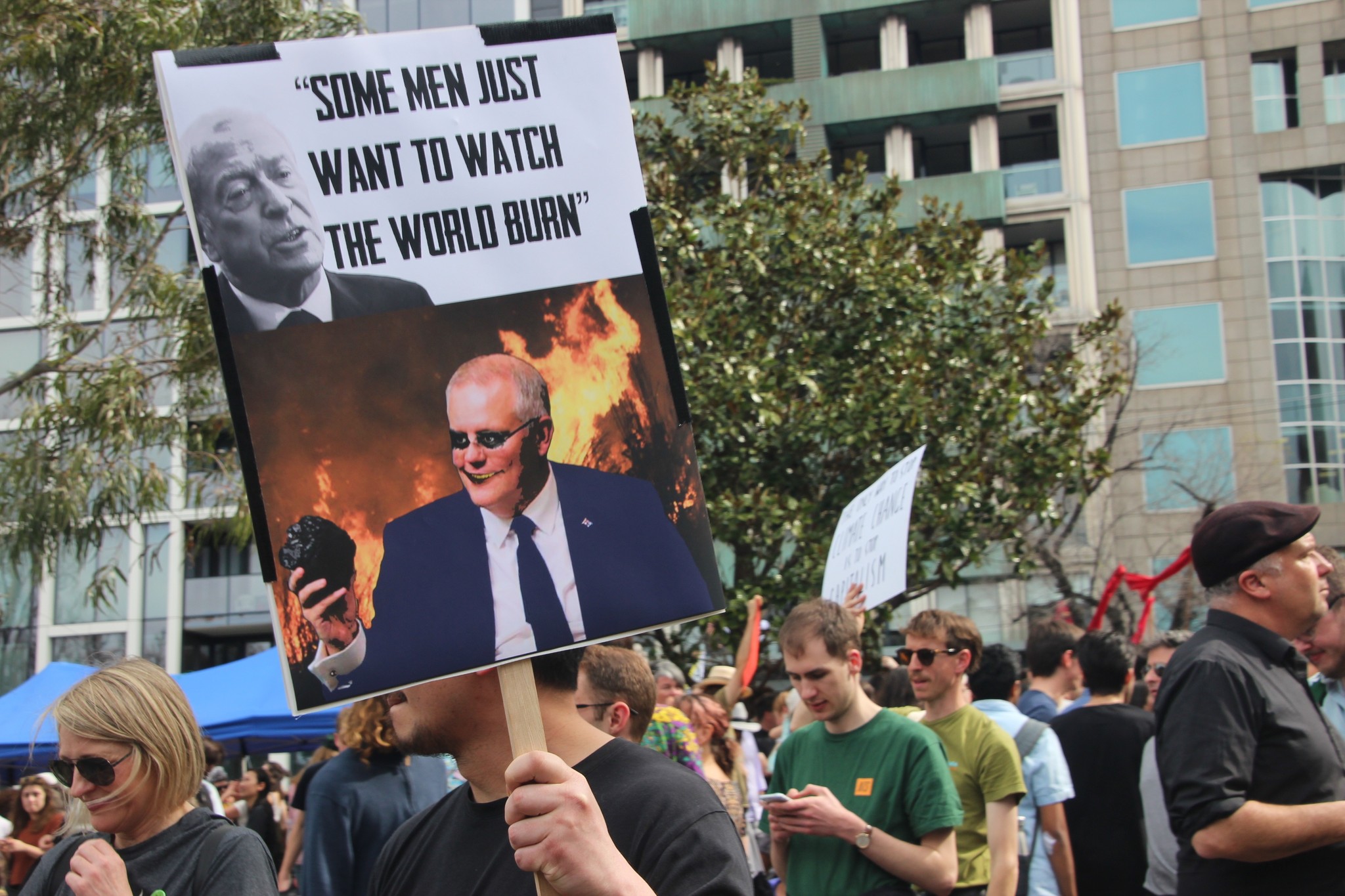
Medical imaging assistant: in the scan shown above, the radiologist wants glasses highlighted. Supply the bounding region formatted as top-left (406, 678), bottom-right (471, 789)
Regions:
top-left (1140, 664), bottom-right (1165, 677)
top-left (895, 649), bottom-right (959, 667)
top-left (1018, 671), bottom-right (1027, 680)
top-left (48, 748), bottom-right (136, 788)
top-left (217, 785), bottom-right (227, 791)
top-left (1298, 594), bottom-right (1345, 643)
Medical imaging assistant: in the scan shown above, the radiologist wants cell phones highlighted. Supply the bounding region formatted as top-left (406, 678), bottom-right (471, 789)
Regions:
top-left (759, 792), bottom-right (792, 803)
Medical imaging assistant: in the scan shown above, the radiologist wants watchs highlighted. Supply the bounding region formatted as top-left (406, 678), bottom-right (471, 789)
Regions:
top-left (854, 824), bottom-right (873, 851)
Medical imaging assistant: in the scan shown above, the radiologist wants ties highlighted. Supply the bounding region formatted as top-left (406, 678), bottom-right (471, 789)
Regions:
top-left (510, 514), bottom-right (574, 652)
top-left (278, 310), bottom-right (319, 336)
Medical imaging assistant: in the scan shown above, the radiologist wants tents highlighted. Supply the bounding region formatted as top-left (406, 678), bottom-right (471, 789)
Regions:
top-left (0, 646), bottom-right (357, 773)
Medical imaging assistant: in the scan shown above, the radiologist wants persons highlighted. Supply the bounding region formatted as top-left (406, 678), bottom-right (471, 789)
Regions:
top-left (0, 503), bottom-right (1345, 896)
top-left (175, 106), bottom-right (438, 336)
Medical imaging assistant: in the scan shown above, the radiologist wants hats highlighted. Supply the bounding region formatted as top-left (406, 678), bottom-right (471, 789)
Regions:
top-left (207, 766), bottom-right (228, 782)
top-left (697, 665), bottom-right (753, 699)
top-left (1191, 501), bottom-right (1320, 588)
top-left (727, 702), bottom-right (762, 733)
